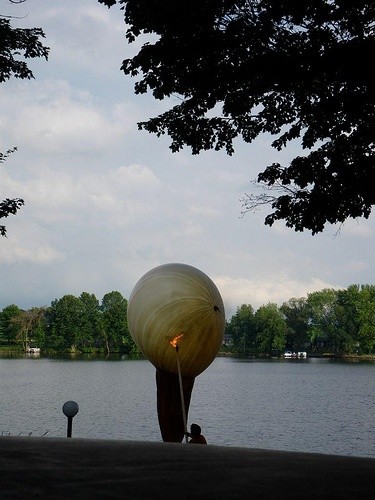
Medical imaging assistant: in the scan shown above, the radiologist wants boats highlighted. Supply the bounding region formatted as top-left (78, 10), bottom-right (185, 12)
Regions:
top-left (28, 344), bottom-right (41, 353)
top-left (282, 351), bottom-right (307, 358)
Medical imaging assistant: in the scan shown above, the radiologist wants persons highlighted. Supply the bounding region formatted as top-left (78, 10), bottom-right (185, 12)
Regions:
top-left (185, 423), bottom-right (207, 445)
